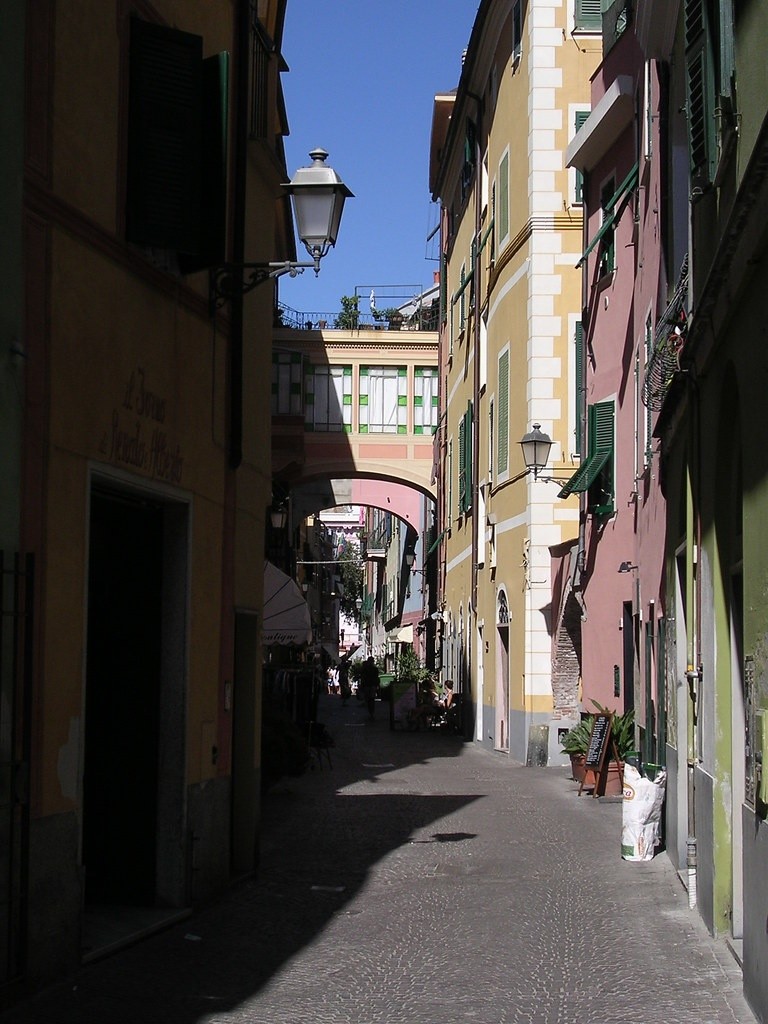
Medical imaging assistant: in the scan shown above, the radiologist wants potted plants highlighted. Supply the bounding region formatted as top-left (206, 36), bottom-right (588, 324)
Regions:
top-left (559, 698), bottom-right (640, 796)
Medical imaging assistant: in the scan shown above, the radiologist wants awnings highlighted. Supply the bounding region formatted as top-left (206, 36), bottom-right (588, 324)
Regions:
top-left (262, 560), bottom-right (312, 645)
top-left (322, 645), bottom-right (342, 665)
top-left (388, 625), bottom-right (415, 643)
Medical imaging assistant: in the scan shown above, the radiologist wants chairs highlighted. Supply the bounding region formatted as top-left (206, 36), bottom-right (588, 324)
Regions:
top-left (429, 694), bottom-right (459, 731)
top-left (310, 723), bottom-right (336, 771)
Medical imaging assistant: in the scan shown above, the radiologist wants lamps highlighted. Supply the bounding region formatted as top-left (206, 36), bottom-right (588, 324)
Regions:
top-left (214, 146), bottom-right (355, 310)
top-left (301, 577), bottom-right (308, 595)
top-left (404, 545), bottom-right (427, 577)
top-left (356, 595), bottom-right (363, 613)
top-left (516, 423), bottom-right (581, 498)
top-left (617, 562), bottom-right (638, 573)
top-left (269, 505), bottom-right (288, 529)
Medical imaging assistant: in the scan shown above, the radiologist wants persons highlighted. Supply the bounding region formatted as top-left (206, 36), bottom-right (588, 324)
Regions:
top-left (435, 680), bottom-right (454, 724)
top-left (327, 666), bottom-right (334, 694)
top-left (421, 680), bottom-right (444, 726)
top-left (360, 656), bottom-right (381, 721)
top-left (333, 666), bottom-right (340, 694)
top-left (335, 656), bottom-right (352, 706)
